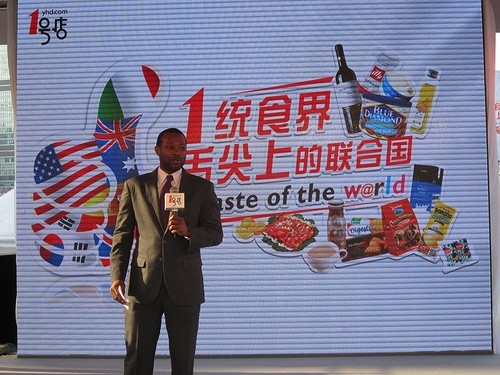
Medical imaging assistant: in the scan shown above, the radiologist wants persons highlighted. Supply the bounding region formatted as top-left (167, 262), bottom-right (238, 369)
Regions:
top-left (110, 128), bottom-right (223, 375)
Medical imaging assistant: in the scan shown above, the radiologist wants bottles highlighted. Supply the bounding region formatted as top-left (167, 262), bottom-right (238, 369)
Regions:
top-left (327, 199), bottom-right (346, 250)
top-left (407, 68), bottom-right (441, 139)
top-left (333, 44), bottom-right (365, 137)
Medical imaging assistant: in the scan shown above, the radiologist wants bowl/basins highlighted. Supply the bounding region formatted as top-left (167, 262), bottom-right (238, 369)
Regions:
top-left (382, 71), bottom-right (415, 102)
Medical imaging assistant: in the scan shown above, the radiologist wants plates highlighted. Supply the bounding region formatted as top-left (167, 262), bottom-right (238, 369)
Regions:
top-left (233, 221), bottom-right (254, 243)
top-left (255, 221), bottom-right (318, 256)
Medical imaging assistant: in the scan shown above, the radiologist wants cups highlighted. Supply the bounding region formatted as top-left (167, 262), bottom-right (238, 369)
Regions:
top-left (301, 241), bottom-right (348, 274)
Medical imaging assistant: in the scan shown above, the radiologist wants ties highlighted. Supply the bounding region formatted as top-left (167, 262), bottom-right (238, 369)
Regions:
top-left (157, 174), bottom-right (175, 232)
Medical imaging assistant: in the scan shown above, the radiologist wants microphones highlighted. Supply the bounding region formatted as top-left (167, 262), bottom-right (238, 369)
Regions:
top-left (164, 186), bottom-right (185, 238)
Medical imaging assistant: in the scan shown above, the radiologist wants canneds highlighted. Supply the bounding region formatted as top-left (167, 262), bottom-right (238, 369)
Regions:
top-left (364, 51), bottom-right (399, 92)
